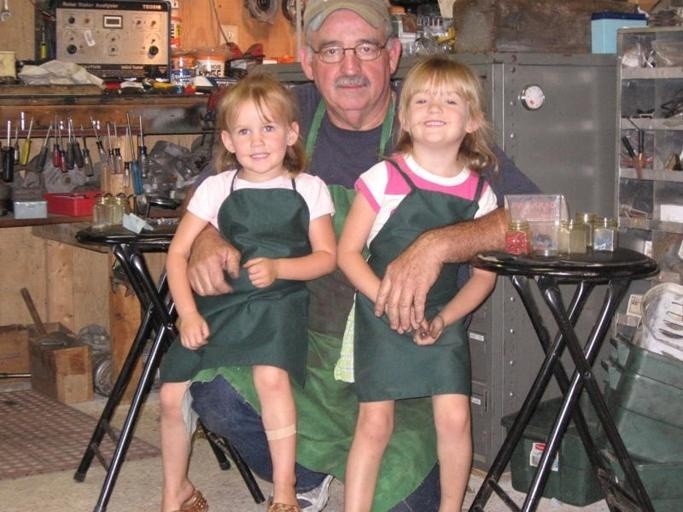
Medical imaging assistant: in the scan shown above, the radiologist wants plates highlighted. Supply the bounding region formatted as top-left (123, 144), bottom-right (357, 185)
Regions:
top-left (32, 223), bottom-right (173, 406)
top-left (0, 84), bottom-right (215, 228)
top-left (619, 26), bottom-right (683, 280)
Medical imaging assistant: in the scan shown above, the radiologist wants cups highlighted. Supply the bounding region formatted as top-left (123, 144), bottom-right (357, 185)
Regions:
top-left (306, 40), bottom-right (388, 63)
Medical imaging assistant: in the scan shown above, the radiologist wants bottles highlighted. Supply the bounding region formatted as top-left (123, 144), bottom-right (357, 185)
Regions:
top-left (304, 0), bottom-right (391, 29)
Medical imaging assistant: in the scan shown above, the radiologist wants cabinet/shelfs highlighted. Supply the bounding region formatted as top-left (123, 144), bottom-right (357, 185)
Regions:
top-left (74, 143), bottom-right (85, 168)
top-left (407, 8), bottom-right (411, 18)
top-left (66, 143), bottom-right (75, 170)
top-left (173, 71), bottom-right (190, 94)
top-left (2, 147), bottom-right (14, 183)
top-left (20, 139), bottom-right (31, 165)
top-left (40, 28), bottom-right (48, 62)
top-left (509, 221), bottom-right (533, 258)
top-left (105, 194), bottom-right (113, 227)
top-left (95, 140), bottom-right (107, 162)
top-left (139, 146), bottom-right (148, 179)
top-left (593, 217), bottom-right (618, 260)
top-left (196, 54), bottom-right (225, 77)
top-left (571, 213), bottom-right (590, 259)
top-left (173, 54), bottom-right (196, 75)
top-left (83, 149), bottom-right (94, 177)
top-left (108, 149), bottom-right (114, 174)
top-left (92, 194), bottom-right (103, 232)
top-left (53, 144), bottom-right (59, 166)
top-left (14, 143), bottom-right (18, 163)
top-left (130, 160), bottom-right (143, 194)
top-left (17, 63), bottom-right (23, 82)
top-left (560, 221), bottom-right (569, 257)
top-left (115, 148), bottom-right (124, 174)
top-left (115, 192), bottom-right (125, 231)
top-left (36, 147), bottom-right (48, 173)
top-left (122, 162), bottom-right (131, 196)
top-left (60, 150), bottom-right (68, 173)
top-left (413, 17), bottom-right (455, 56)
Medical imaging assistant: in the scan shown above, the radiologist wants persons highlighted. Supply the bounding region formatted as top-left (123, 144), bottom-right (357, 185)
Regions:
top-left (338, 58), bottom-right (499, 512)
top-left (182, 0), bottom-right (544, 512)
top-left (158, 73), bottom-right (337, 512)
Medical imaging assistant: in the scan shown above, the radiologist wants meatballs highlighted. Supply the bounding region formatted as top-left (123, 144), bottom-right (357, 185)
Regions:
top-left (39, 26), bottom-right (48, 61)
top-left (4, 121), bottom-right (14, 183)
top-left (72, 124), bottom-right (83, 168)
top-left (66, 118), bottom-right (73, 169)
top-left (108, 124), bottom-right (117, 173)
top-left (90, 115), bottom-right (107, 160)
top-left (80, 123), bottom-right (94, 176)
top-left (34, 125), bottom-right (52, 172)
top-left (139, 116), bottom-right (147, 179)
top-left (59, 127), bottom-right (66, 175)
top-left (21, 113), bottom-right (35, 166)
top-left (114, 122), bottom-right (123, 174)
top-left (54, 112), bottom-right (60, 165)
top-left (15, 127), bottom-right (19, 167)
top-left (127, 113), bottom-right (143, 194)
top-left (124, 128), bottom-right (130, 189)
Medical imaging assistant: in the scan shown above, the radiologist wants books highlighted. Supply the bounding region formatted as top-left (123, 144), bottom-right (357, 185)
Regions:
top-left (0, 324), bottom-right (32, 376)
top-left (29, 321), bottom-right (94, 404)
top-left (600, 330), bottom-right (682, 465)
top-left (499, 399), bottom-right (617, 508)
top-left (631, 282), bottom-right (682, 362)
top-left (250, 52), bottom-right (622, 475)
top-left (592, 12), bottom-right (648, 55)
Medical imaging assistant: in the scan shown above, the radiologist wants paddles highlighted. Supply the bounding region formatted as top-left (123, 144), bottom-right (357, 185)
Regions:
top-left (1, 388), bottom-right (163, 479)
